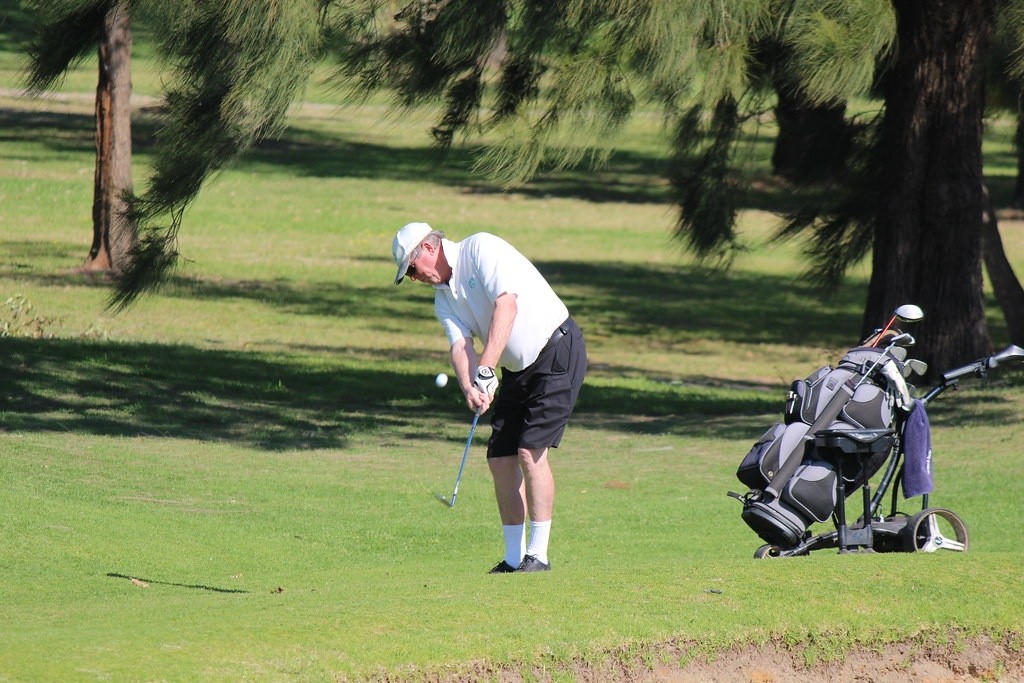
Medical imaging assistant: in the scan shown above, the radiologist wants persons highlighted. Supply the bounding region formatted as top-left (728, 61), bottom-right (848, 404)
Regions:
top-left (393, 223), bottom-right (587, 573)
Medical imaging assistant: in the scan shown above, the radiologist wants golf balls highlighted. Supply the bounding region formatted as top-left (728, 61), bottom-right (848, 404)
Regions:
top-left (436, 373), bottom-right (448, 388)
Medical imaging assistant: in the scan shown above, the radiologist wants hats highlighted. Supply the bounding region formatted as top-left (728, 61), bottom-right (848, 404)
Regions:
top-left (392, 222), bottom-right (432, 283)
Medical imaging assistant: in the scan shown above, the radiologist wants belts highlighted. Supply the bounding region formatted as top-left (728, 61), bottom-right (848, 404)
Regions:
top-left (501, 312), bottom-right (573, 378)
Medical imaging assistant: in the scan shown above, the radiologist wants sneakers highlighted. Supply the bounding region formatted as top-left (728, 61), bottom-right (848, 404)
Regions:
top-left (488, 560), bottom-right (518, 574)
top-left (516, 554), bottom-right (551, 572)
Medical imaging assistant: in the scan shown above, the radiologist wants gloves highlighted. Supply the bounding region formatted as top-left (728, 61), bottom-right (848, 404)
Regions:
top-left (879, 358), bottom-right (909, 408)
top-left (473, 366), bottom-right (500, 397)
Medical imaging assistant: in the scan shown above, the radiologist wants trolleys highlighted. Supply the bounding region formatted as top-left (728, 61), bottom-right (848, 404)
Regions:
top-left (727, 329), bottom-right (1024, 559)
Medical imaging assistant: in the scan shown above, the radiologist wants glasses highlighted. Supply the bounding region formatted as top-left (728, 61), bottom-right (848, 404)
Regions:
top-left (395, 251), bottom-right (420, 286)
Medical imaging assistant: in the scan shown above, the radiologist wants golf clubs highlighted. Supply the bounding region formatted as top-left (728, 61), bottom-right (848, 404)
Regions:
top-left (431, 400), bottom-right (483, 507)
top-left (861, 303), bottom-right (929, 398)
